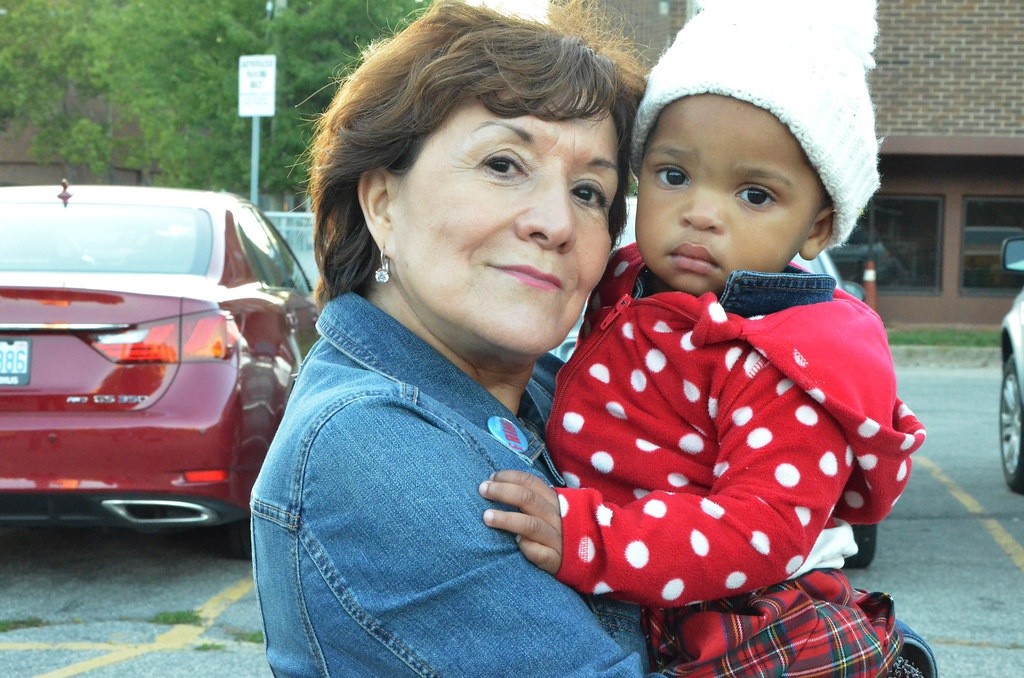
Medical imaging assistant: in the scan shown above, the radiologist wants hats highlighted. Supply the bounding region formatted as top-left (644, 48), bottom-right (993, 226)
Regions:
top-left (630, 0), bottom-right (881, 250)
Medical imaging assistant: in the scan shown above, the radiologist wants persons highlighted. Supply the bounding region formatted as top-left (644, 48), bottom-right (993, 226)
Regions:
top-left (248, 0), bottom-right (937, 678)
top-left (479, 0), bottom-right (926, 678)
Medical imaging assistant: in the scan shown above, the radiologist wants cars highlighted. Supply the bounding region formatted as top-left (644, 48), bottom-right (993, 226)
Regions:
top-left (1, 182), bottom-right (317, 558)
top-left (830, 217), bottom-right (892, 289)
top-left (541, 192), bottom-right (869, 367)
top-left (962, 227), bottom-right (1024, 290)
top-left (997, 237), bottom-right (1024, 493)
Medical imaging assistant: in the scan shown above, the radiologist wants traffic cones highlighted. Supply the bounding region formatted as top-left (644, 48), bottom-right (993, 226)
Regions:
top-left (862, 256), bottom-right (876, 312)
